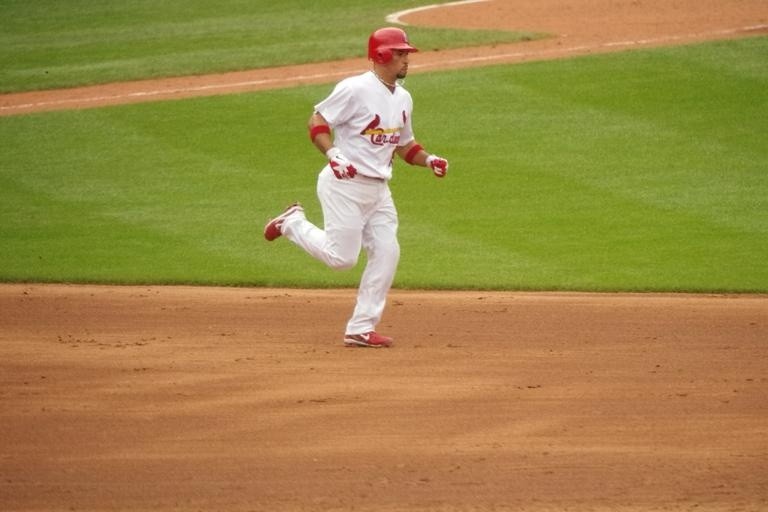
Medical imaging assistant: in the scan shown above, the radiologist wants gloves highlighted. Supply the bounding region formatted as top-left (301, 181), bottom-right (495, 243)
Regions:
top-left (424, 153), bottom-right (448, 177)
top-left (326, 147), bottom-right (357, 180)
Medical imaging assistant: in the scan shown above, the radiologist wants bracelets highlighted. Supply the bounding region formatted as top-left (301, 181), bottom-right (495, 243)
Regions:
top-left (424, 154), bottom-right (438, 166)
top-left (325, 147), bottom-right (342, 160)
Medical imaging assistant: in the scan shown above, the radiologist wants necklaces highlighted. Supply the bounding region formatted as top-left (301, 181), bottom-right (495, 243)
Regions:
top-left (371, 67), bottom-right (406, 88)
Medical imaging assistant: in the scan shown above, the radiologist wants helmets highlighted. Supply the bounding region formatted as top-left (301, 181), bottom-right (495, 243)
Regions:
top-left (368, 27), bottom-right (418, 65)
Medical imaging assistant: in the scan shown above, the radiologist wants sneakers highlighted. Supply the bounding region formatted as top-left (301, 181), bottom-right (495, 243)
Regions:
top-left (264, 202), bottom-right (304, 241)
top-left (344, 330), bottom-right (394, 349)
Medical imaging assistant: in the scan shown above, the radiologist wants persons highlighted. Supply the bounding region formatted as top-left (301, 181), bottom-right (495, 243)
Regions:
top-left (263, 25), bottom-right (450, 349)
